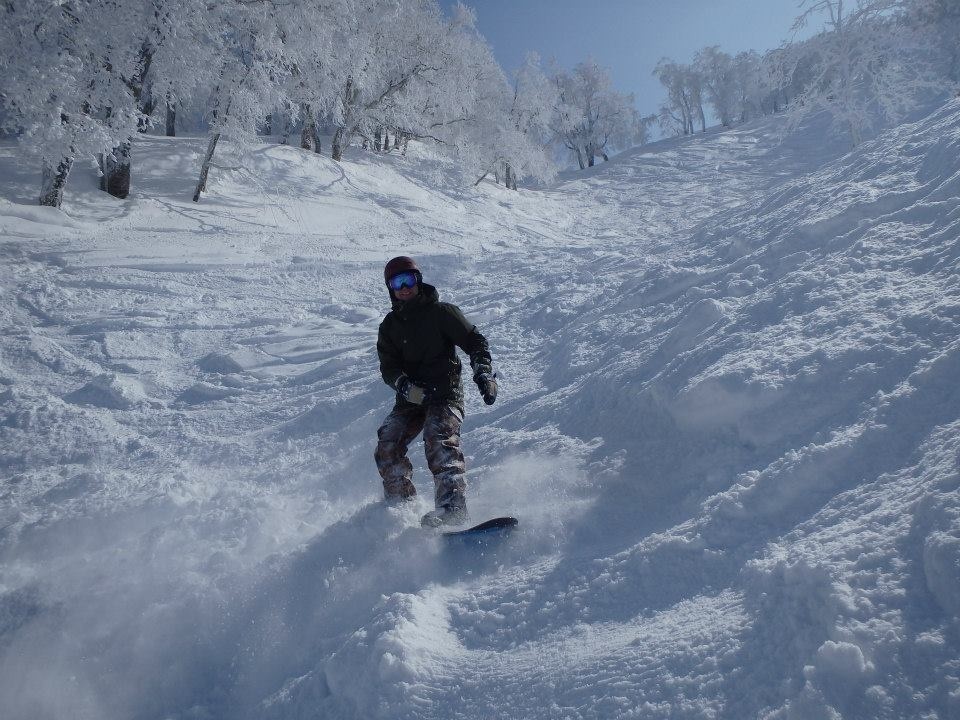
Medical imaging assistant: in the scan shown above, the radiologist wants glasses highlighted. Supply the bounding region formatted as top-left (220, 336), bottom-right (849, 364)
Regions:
top-left (385, 273), bottom-right (420, 290)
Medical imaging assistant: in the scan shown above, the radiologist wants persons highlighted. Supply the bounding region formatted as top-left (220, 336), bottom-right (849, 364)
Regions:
top-left (374, 256), bottom-right (498, 528)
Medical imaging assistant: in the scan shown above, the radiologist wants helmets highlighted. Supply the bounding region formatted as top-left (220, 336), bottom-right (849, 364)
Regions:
top-left (384, 256), bottom-right (423, 282)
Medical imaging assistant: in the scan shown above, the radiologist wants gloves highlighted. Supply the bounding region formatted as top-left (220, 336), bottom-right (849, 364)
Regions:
top-left (473, 373), bottom-right (499, 405)
top-left (395, 375), bottom-right (438, 406)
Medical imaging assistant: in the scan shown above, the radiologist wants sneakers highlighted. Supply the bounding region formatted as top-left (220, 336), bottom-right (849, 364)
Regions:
top-left (419, 504), bottom-right (472, 533)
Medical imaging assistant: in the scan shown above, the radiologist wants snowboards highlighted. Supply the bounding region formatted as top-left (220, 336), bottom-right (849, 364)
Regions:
top-left (443, 517), bottom-right (518, 536)
